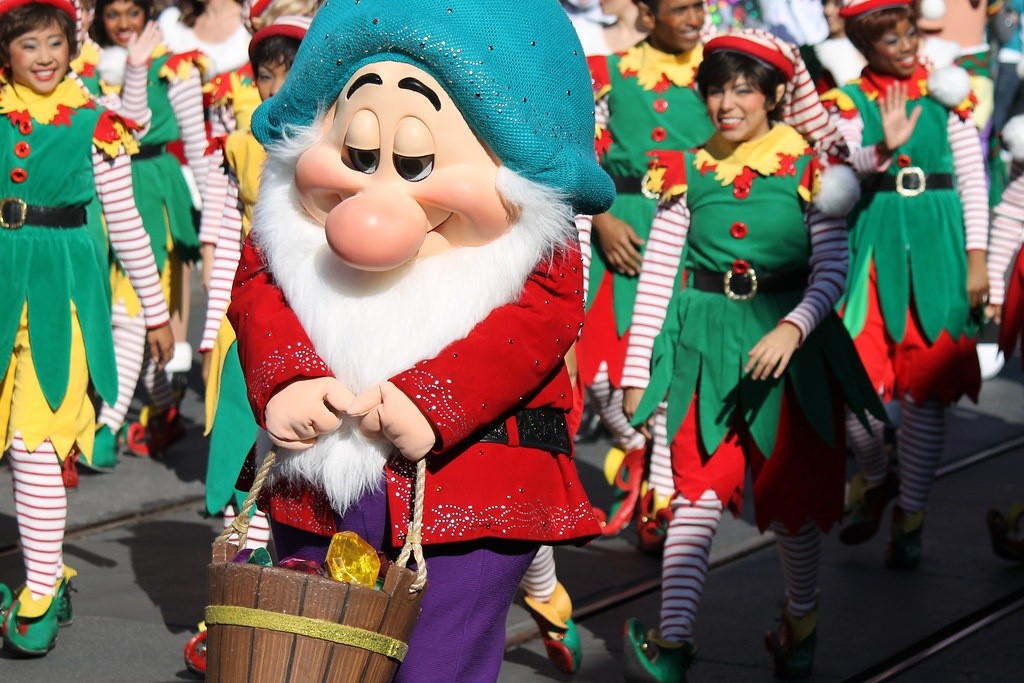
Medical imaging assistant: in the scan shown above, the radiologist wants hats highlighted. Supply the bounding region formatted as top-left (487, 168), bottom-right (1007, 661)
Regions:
top-left (0, 0), bottom-right (82, 24)
top-left (247, 13), bottom-right (314, 55)
top-left (838, 0), bottom-right (913, 19)
top-left (701, 26), bottom-right (852, 163)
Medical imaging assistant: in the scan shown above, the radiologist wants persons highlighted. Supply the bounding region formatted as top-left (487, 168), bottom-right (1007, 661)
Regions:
top-left (226, 0), bottom-right (615, 683)
top-left (514, 0), bottom-right (1024, 683)
top-left (0, 0), bottom-right (329, 653)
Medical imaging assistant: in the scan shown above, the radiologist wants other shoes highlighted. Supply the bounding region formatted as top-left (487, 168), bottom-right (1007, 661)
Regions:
top-left (524, 580), bottom-right (583, 675)
top-left (837, 473), bottom-right (898, 551)
top-left (637, 483), bottom-right (667, 558)
top-left (126, 402), bottom-right (186, 460)
top-left (73, 422), bottom-right (131, 473)
top-left (0, 575), bottom-right (69, 656)
top-left (987, 500), bottom-right (1024, 564)
top-left (620, 616), bottom-right (698, 683)
top-left (765, 588), bottom-right (821, 683)
top-left (53, 563), bottom-right (76, 627)
top-left (884, 505), bottom-right (927, 574)
top-left (592, 439), bottom-right (649, 537)
top-left (183, 621), bottom-right (209, 677)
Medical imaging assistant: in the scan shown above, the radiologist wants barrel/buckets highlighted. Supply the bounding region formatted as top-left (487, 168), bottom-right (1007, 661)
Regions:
top-left (205, 443), bottom-right (430, 683)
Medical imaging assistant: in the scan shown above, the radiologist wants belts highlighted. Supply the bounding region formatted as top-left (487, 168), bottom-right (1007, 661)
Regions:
top-left (611, 169), bottom-right (666, 201)
top-left (128, 142), bottom-right (169, 162)
top-left (864, 168), bottom-right (956, 197)
top-left (691, 267), bottom-right (810, 301)
top-left (0, 195), bottom-right (89, 229)
